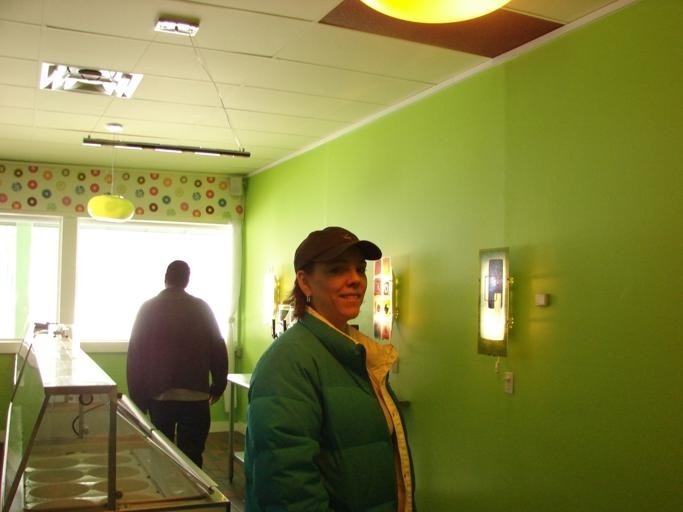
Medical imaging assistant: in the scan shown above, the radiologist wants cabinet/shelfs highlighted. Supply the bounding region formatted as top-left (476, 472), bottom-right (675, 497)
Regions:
top-left (0, 321), bottom-right (233, 509)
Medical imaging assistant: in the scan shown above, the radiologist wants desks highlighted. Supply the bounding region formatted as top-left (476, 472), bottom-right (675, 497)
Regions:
top-left (220, 371), bottom-right (412, 484)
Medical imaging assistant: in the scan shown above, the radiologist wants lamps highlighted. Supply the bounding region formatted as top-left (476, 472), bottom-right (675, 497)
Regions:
top-left (80, 17), bottom-right (253, 161)
top-left (80, 143), bottom-right (139, 222)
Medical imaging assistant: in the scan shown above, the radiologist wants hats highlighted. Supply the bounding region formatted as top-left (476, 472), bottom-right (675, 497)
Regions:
top-left (294, 225), bottom-right (382, 273)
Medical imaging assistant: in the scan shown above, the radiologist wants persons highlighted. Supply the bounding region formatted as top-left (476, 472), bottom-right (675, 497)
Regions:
top-left (241, 226), bottom-right (416, 511)
top-left (126, 260), bottom-right (228, 470)
